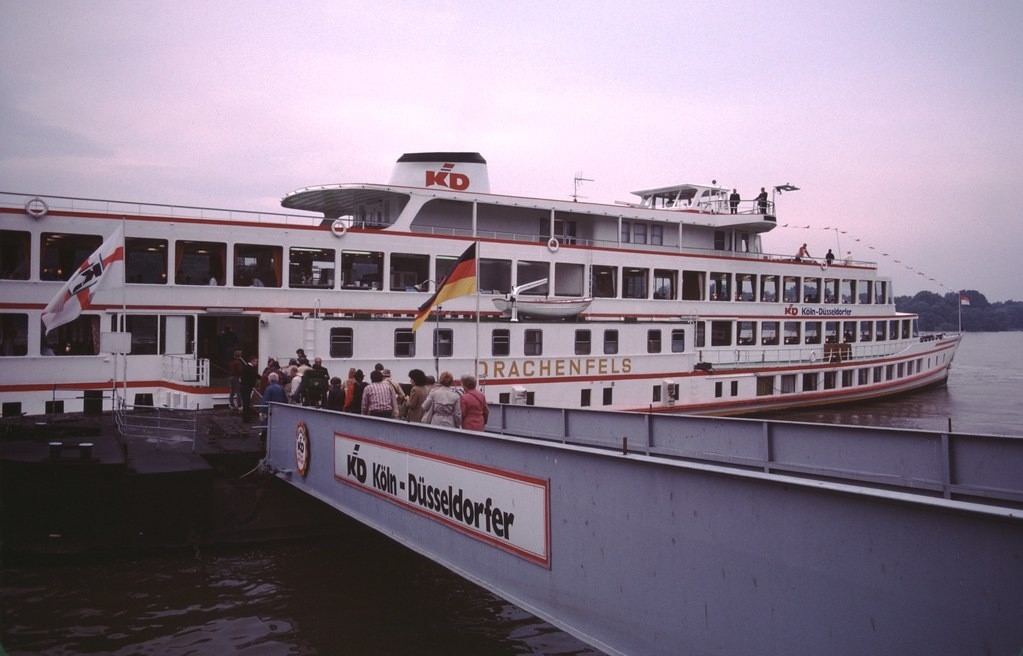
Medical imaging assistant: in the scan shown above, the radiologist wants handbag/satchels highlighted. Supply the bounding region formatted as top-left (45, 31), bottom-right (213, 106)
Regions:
top-left (399, 400), bottom-right (409, 418)
top-left (422, 401), bottom-right (435, 424)
top-left (483, 405), bottom-right (489, 424)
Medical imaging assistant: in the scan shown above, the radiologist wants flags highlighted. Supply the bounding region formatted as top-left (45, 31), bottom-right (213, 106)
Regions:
top-left (42, 222), bottom-right (124, 337)
top-left (960, 295), bottom-right (970, 305)
top-left (412, 240), bottom-right (477, 334)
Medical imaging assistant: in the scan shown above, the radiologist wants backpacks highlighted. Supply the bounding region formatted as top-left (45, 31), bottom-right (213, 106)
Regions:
top-left (302, 374), bottom-right (326, 406)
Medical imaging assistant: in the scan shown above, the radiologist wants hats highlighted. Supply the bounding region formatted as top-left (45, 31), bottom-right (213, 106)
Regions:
top-left (381, 368), bottom-right (391, 377)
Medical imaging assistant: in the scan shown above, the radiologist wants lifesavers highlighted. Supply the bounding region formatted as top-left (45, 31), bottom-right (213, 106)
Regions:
top-left (810, 350), bottom-right (816, 362)
top-left (821, 260), bottom-right (828, 270)
top-left (25, 197), bottom-right (49, 218)
top-left (332, 219), bottom-right (347, 238)
top-left (547, 238), bottom-right (560, 251)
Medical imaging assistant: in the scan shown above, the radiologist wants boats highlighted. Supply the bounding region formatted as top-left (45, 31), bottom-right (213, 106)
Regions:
top-left (491, 296), bottom-right (595, 316)
top-left (1, 152), bottom-right (963, 547)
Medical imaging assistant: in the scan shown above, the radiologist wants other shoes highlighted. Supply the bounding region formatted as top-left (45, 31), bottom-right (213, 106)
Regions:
top-left (231, 406), bottom-right (238, 409)
top-left (238, 406), bottom-right (243, 410)
top-left (257, 437), bottom-right (265, 444)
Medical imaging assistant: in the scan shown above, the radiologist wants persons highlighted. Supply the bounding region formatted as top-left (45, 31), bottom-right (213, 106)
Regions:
top-left (753, 187), bottom-right (767, 215)
top-left (796, 244), bottom-right (811, 263)
top-left (207, 274), bottom-right (218, 286)
top-left (844, 330), bottom-right (850, 338)
top-left (847, 252), bottom-right (853, 266)
top-left (249, 276), bottom-right (265, 287)
top-left (229, 347), bottom-right (491, 452)
top-left (729, 188), bottom-right (740, 214)
top-left (826, 249), bottom-right (835, 265)
top-left (41, 342), bottom-right (56, 356)
